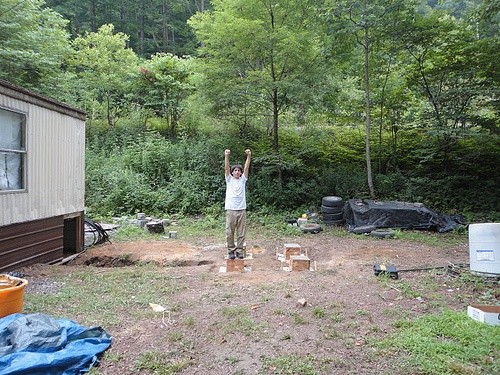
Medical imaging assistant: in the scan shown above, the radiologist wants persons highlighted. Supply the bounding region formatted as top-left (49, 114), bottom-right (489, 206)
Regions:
top-left (222, 148), bottom-right (252, 260)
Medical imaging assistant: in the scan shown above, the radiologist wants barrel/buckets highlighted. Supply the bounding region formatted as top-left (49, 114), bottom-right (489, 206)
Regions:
top-left (468, 223), bottom-right (500, 278)
top-left (0, 277), bottom-right (28, 318)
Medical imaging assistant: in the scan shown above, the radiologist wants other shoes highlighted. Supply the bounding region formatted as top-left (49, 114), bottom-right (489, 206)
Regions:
top-left (236, 252), bottom-right (243, 259)
top-left (228, 252), bottom-right (235, 259)
